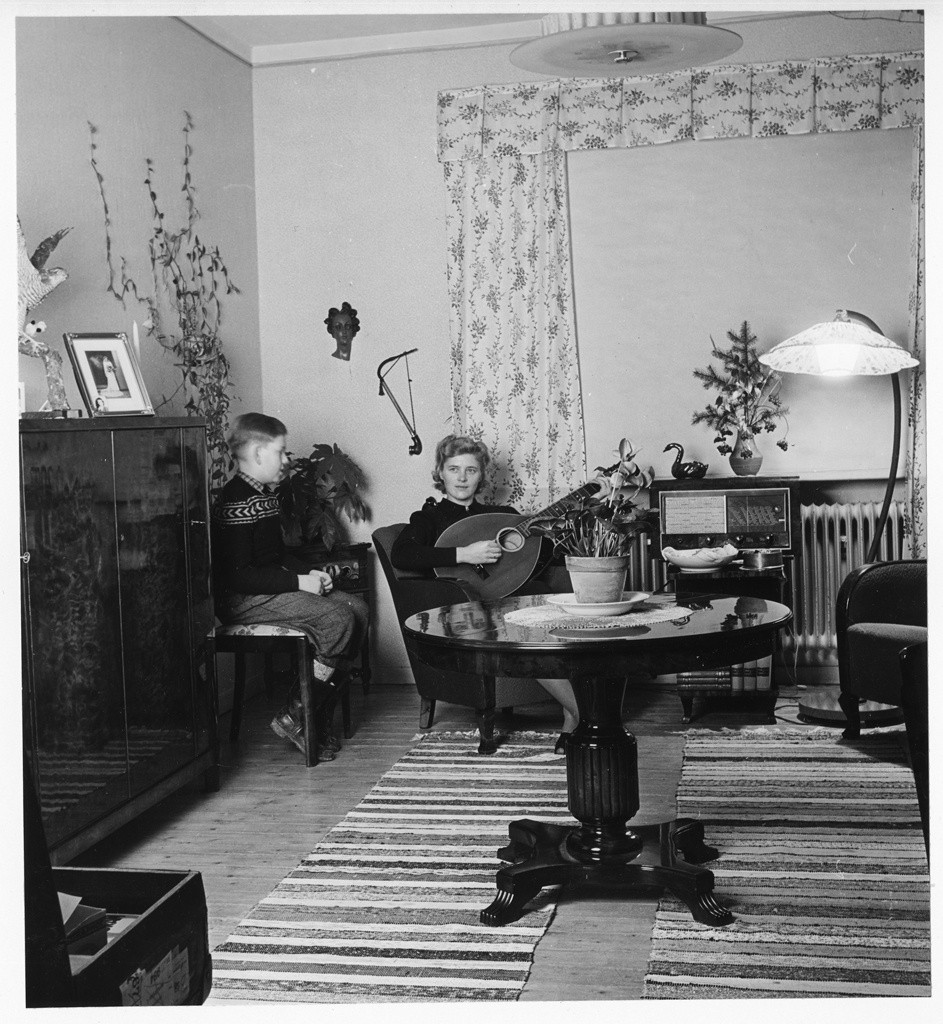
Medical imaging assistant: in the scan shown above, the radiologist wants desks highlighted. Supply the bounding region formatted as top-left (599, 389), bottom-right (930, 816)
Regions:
top-left (298, 541), bottom-right (371, 697)
top-left (406, 592), bottom-right (793, 926)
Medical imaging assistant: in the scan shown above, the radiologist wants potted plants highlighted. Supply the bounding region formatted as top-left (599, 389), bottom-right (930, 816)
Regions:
top-left (274, 443), bottom-right (372, 549)
top-left (525, 437), bottom-right (656, 614)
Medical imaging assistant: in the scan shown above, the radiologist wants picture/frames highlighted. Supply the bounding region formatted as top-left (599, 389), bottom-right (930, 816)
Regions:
top-left (63, 330), bottom-right (158, 419)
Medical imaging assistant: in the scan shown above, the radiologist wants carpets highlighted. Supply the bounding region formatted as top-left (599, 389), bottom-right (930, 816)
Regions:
top-left (642, 728), bottom-right (932, 999)
top-left (200, 731), bottom-right (581, 1006)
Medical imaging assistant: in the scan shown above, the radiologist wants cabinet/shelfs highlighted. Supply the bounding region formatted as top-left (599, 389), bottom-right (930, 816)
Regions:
top-left (20, 415), bottom-right (220, 865)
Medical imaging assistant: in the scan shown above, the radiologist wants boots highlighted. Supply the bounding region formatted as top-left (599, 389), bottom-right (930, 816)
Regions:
top-left (317, 691), bottom-right (342, 751)
top-left (269, 672), bottom-right (339, 761)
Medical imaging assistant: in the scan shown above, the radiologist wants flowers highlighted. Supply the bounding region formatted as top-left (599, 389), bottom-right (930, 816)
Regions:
top-left (691, 320), bottom-right (790, 460)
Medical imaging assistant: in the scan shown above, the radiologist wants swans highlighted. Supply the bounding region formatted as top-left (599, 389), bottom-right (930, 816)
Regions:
top-left (663, 443), bottom-right (709, 480)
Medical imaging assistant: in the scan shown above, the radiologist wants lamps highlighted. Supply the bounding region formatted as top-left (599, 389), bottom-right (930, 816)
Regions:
top-left (758, 309), bottom-right (920, 721)
top-left (509, 11), bottom-right (743, 77)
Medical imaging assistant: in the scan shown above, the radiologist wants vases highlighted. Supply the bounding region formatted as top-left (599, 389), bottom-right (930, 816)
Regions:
top-left (728, 427), bottom-right (762, 476)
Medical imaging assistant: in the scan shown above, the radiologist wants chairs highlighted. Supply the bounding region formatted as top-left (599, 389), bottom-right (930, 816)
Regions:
top-left (836, 558), bottom-right (934, 739)
top-left (208, 568), bottom-right (356, 766)
top-left (371, 521), bottom-right (575, 756)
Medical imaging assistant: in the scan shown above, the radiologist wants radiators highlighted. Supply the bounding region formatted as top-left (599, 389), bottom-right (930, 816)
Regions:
top-left (630, 498), bottom-right (903, 685)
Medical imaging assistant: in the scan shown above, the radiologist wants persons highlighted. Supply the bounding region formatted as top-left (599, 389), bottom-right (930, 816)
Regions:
top-left (209, 413), bottom-right (369, 761)
top-left (390, 435), bottom-right (580, 754)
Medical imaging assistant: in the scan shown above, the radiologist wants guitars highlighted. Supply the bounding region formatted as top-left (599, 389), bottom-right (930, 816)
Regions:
top-left (433, 452), bottom-right (638, 601)
